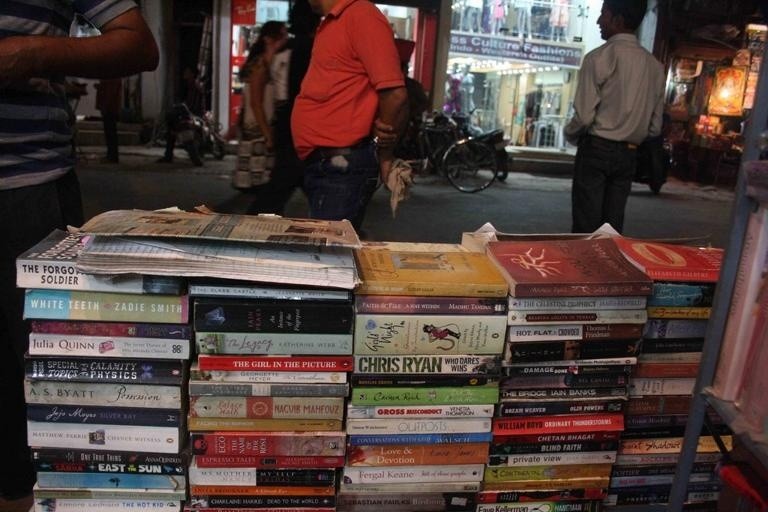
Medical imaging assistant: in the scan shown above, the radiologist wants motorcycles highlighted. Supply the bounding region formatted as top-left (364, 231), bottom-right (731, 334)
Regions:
top-left (632, 113), bottom-right (671, 193)
top-left (447, 105), bottom-right (511, 181)
top-left (171, 77), bottom-right (226, 166)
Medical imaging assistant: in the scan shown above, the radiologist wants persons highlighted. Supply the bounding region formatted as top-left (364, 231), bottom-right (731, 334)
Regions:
top-left (563, 0), bottom-right (667, 234)
top-left (442, 59), bottom-right (478, 125)
top-left (0, 0), bottom-right (162, 511)
top-left (93, 77), bottom-right (123, 164)
top-left (524, 80), bottom-right (550, 144)
top-left (157, 62), bottom-right (212, 165)
top-left (233, 0), bottom-right (426, 237)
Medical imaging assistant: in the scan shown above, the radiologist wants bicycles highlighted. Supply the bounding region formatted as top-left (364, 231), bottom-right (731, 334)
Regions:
top-left (403, 121), bottom-right (501, 193)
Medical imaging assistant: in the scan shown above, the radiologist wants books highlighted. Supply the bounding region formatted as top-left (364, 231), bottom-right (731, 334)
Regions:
top-left (17, 204), bottom-right (767, 511)
